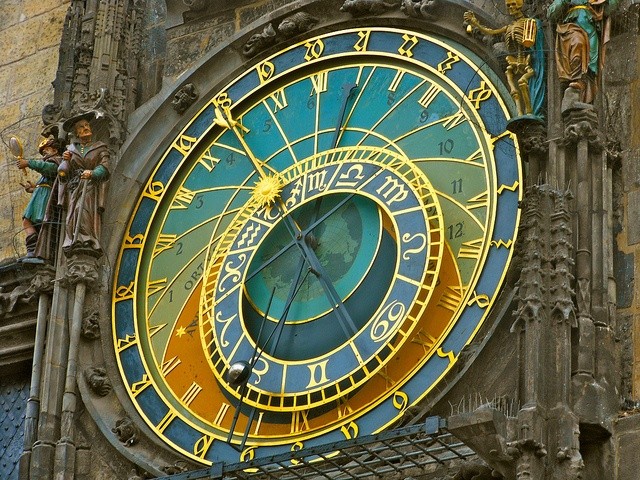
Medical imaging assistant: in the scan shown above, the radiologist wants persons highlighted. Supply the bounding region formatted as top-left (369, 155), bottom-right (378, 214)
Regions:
top-left (56, 112), bottom-right (110, 250)
top-left (7, 136), bottom-right (60, 265)
top-left (545, 0), bottom-right (618, 116)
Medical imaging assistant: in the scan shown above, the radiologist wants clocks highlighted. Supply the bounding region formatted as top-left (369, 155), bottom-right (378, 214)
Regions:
top-left (108, 25), bottom-right (524, 475)
